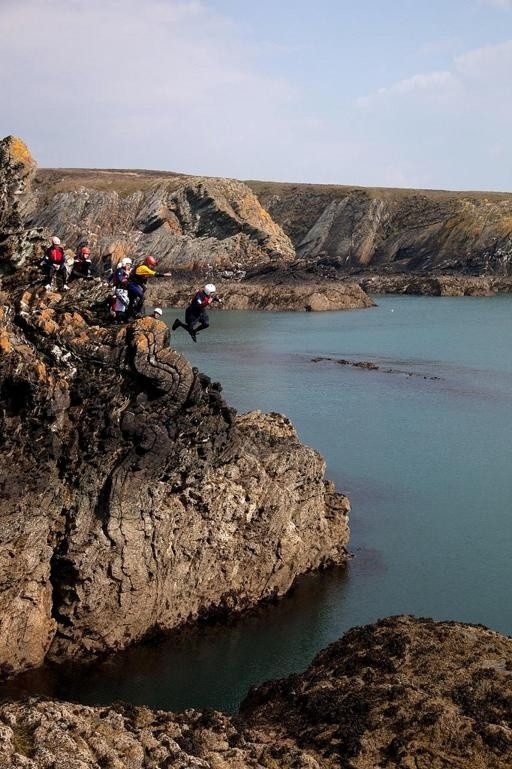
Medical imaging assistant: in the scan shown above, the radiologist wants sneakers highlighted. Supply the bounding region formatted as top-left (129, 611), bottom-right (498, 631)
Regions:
top-left (63, 285), bottom-right (70, 290)
top-left (44, 284), bottom-right (51, 290)
top-left (190, 330), bottom-right (197, 342)
top-left (172, 319), bottom-right (179, 330)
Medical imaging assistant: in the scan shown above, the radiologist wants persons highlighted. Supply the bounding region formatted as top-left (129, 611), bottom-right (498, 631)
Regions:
top-left (171, 282), bottom-right (226, 342)
top-left (42, 237), bottom-right (174, 320)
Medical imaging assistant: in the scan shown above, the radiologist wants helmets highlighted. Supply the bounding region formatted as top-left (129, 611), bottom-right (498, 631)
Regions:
top-left (122, 258), bottom-right (132, 268)
top-left (81, 247), bottom-right (90, 254)
top-left (145, 256), bottom-right (156, 267)
top-left (155, 307), bottom-right (163, 315)
top-left (204, 283), bottom-right (215, 296)
top-left (52, 236), bottom-right (60, 245)
